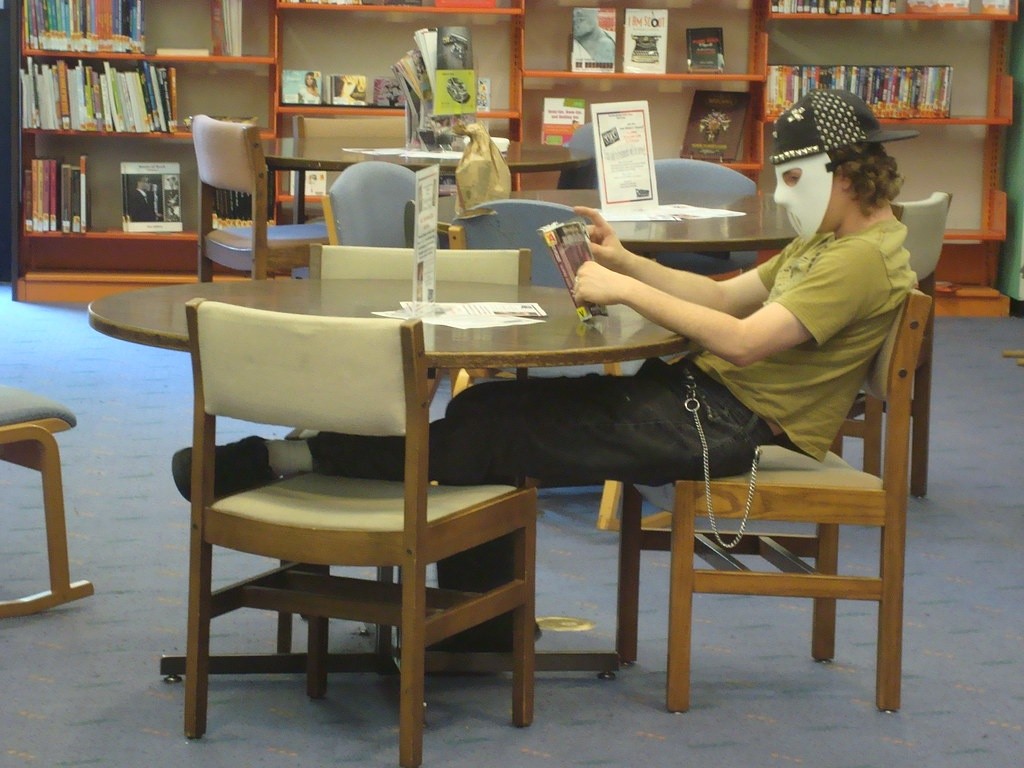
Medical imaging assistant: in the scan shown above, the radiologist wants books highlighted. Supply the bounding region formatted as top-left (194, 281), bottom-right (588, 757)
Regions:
top-left (210, 168), bottom-right (277, 234)
top-left (621, 5), bottom-right (670, 75)
top-left (119, 159), bottom-right (183, 234)
top-left (19, 1), bottom-right (262, 136)
top-left (765, 0), bottom-right (1015, 121)
top-left (538, 94), bottom-right (587, 153)
top-left (21, 152), bottom-right (92, 235)
top-left (567, 5), bottom-right (618, 74)
top-left (280, 0), bottom-right (498, 198)
top-left (680, 86), bottom-right (752, 165)
top-left (684, 26), bottom-right (726, 75)
top-left (534, 216), bottom-right (612, 323)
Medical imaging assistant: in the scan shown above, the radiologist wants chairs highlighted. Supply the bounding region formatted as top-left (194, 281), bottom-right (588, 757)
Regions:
top-left (320, 122), bottom-right (760, 287)
top-left (830, 188), bottom-right (953, 499)
top-left (0, 382), bottom-right (95, 620)
top-left (183, 295), bottom-right (538, 768)
top-left (276, 241), bottom-right (534, 654)
top-left (612, 289), bottom-right (934, 716)
top-left (191, 113), bottom-right (330, 284)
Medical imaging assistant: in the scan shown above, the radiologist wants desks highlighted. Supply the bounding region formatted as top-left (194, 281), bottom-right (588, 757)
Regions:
top-left (88, 278), bottom-right (694, 686)
top-left (257, 135), bottom-right (594, 226)
top-left (402, 189), bottom-right (802, 253)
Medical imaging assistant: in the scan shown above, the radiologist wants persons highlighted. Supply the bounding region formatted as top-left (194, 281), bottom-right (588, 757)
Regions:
top-left (129, 174), bottom-right (162, 223)
top-left (334, 76), bottom-right (366, 105)
top-left (571, 7), bottom-right (616, 73)
top-left (167, 81), bottom-right (924, 680)
top-left (297, 72), bottom-right (322, 105)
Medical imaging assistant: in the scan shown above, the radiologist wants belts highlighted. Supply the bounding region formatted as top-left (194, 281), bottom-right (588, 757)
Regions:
top-left (649, 359), bottom-right (772, 445)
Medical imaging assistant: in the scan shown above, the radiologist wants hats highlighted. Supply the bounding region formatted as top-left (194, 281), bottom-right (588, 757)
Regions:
top-left (770, 90), bottom-right (920, 166)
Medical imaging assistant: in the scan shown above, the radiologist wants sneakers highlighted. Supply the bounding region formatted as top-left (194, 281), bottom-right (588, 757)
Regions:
top-left (172, 436), bottom-right (276, 502)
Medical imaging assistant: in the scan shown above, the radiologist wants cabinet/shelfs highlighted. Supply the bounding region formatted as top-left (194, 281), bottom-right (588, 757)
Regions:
top-left (12, 0), bottom-right (1022, 318)
